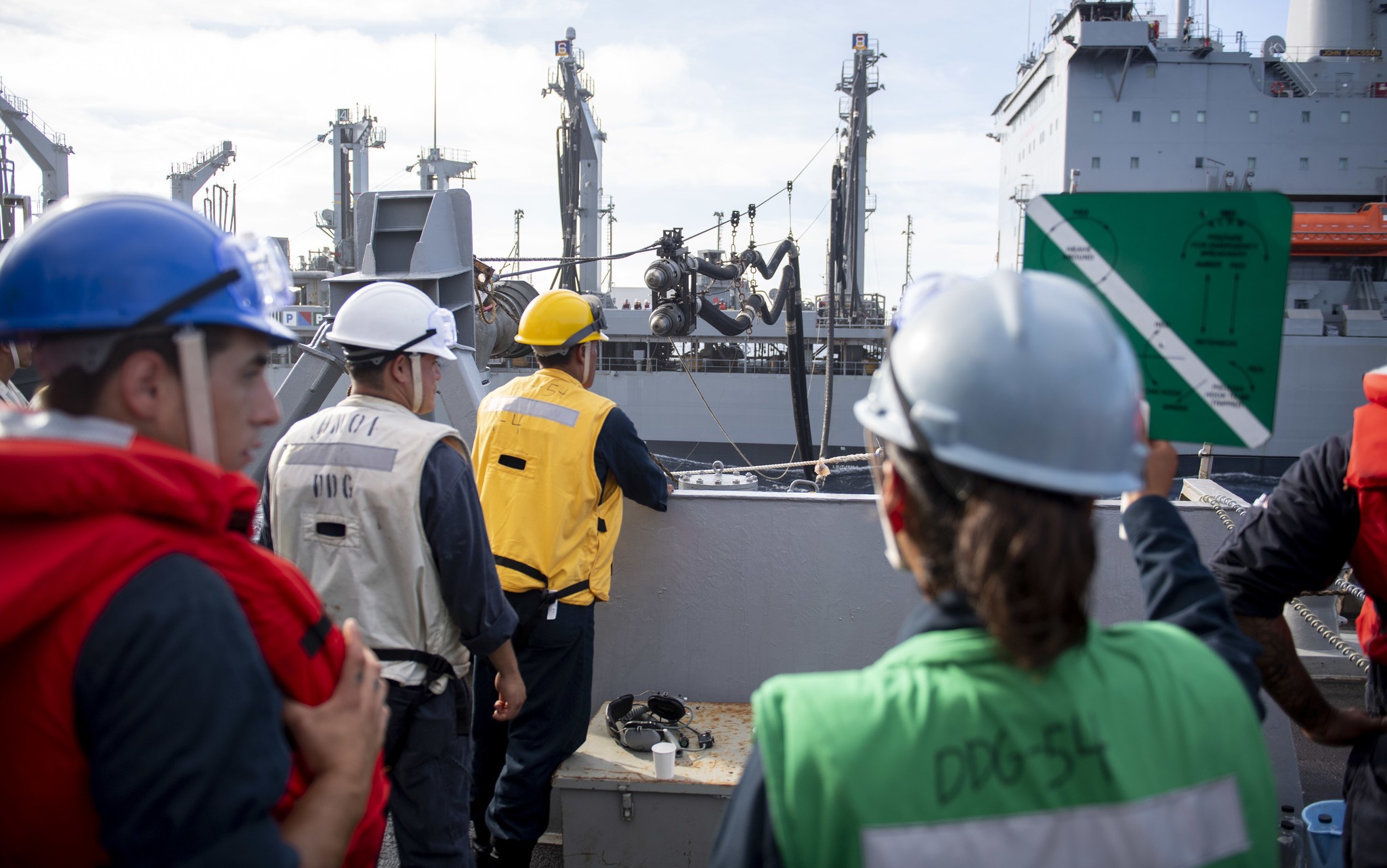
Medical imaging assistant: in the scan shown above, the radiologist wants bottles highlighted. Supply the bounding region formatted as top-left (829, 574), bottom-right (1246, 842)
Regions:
top-left (1276, 800), bottom-right (1342, 868)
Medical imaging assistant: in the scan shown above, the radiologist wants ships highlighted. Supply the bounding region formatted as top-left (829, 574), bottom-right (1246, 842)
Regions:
top-left (0, 1), bottom-right (1387, 508)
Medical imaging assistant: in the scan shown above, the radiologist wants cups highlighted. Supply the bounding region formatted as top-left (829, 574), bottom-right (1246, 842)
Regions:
top-left (651, 742), bottom-right (676, 779)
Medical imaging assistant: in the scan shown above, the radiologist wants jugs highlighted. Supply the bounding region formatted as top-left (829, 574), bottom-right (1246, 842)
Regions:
top-left (1301, 800), bottom-right (1345, 868)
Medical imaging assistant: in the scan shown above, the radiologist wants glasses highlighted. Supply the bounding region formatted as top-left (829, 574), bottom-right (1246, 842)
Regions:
top-left (214, 230), bottom-right (295, 313)
top-left (588, 302), bottom-right (608, 333)
top-left (428, 307), bottom-right (458, 348)
top-left (892, 272), bottom-right (975, 328)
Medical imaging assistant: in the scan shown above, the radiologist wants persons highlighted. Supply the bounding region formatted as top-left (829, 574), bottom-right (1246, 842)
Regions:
top-left (714, 268), bottom-right (1285, 868)
top-left (1217, 365), bottom-right (1386, 868)
top-left (256, 282), bottom-right (530, 867)
top-left (0, 194), bottom-right (389, 868)
top-left (466, 292), bottom-right (671, 867)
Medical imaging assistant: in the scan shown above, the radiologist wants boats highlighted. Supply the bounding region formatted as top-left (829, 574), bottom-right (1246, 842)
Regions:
top-left (1286, 201), bottom-right (1387, 258)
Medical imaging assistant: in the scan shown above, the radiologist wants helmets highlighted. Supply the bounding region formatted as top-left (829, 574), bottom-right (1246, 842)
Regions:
top-left (514, 289), bottom-right (609, 345)
top-left (0, 194), bottom-right (300, 344)
top-left (325, 281), bottom-right (458, 361)
top-left (854, 269), bottom-right (1150, 496)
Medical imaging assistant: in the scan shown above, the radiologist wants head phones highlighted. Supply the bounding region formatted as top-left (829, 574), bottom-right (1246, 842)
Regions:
top-left (604, 690), bottom-right (693, 752)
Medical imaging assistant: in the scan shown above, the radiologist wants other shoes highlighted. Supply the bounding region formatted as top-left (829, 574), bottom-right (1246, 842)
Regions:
top-left (471, 837), bottom-right (532, 868)
